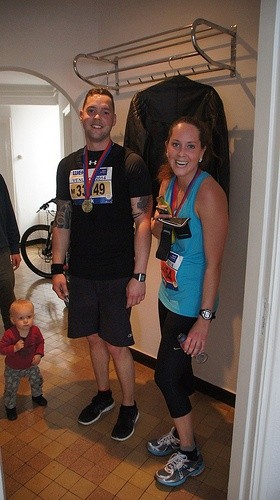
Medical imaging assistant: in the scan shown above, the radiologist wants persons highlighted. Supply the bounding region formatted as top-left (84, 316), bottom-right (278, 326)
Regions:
top-left (0, 298), bottom-right (47, 421)
top-left (51, 87), bottom-right (152, 441)
top-left (0, 173), bottom-right (21, 331)
top-left (147, 116), bottom-right (227, 486)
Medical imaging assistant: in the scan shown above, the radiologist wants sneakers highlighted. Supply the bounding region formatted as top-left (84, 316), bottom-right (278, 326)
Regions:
top-left (148, 426), bottom-right (197, 455)
top-left (154, 448), bottom-right (206, 486)
top-left (77, 391), bottom-right (115, 425)
top-left (111, 402), bottom-right (140, 441)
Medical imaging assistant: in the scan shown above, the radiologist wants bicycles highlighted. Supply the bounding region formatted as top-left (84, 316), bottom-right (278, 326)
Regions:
top-left (21, 198), bottom-right (66, 279)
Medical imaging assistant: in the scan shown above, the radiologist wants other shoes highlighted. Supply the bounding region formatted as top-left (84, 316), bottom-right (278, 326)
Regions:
top-left (32, 394), bottom-right (47, 406)
top-left (5, 406), bottom-right (17, 419)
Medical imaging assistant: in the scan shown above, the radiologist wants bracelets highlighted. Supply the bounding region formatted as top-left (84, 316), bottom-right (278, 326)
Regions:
top-left (51, 264), bottom-right (64, 275)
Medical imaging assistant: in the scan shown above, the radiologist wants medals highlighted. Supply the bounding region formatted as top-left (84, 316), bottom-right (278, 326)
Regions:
top-left (158, 200), bottom-right (172, 215)
top-left (82, 200), bottom-right (92, 212)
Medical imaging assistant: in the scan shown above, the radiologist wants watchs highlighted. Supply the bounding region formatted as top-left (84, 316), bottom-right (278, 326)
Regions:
top-left (133, 273), bottom-right (146, 282)
top-left (199, 308), bottom-right (216, 320)
